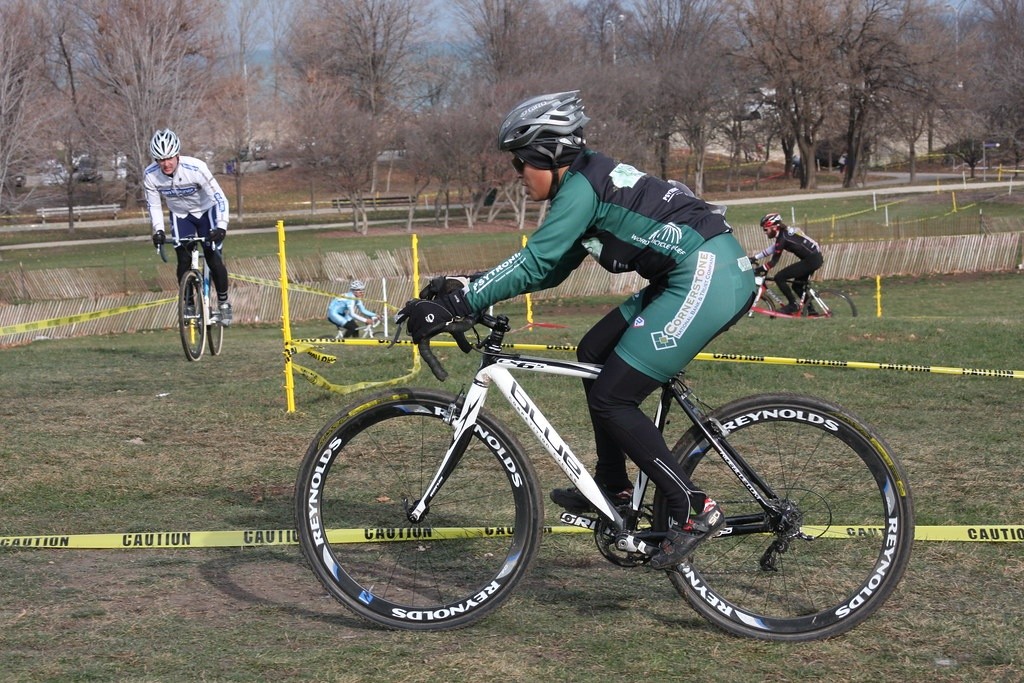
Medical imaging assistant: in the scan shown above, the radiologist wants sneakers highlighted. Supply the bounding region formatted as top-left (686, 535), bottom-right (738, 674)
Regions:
top-left (218, 300), bottom-right (232, 320)
top-left (550, 484), bottom-right (632, 510)
top-left (650, 497), bottom-right (725, 571)
top-left (184, 306), bottom-right (196, 318)
top-left (777, 301), bottom-right (799, 314)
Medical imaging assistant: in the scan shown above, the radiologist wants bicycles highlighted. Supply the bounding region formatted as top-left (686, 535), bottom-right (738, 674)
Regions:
top-left (744, 257), bottom-right (857, 318)
top-left (318, 316), bottom-right (392, 339)
top-left (156, 231), bottom-right (223, 361)
top-left (293, 299), bottom-right (917, 643)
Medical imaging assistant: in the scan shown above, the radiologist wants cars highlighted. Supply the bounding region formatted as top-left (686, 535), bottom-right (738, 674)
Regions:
top-left (15, 153), bottom-right (291, 188)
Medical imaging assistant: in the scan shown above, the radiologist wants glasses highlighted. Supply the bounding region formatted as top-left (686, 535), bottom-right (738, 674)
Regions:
top-left (763, 229), bottom-right (769, 231)
top-left (512, 156), bottom-right (526, 175)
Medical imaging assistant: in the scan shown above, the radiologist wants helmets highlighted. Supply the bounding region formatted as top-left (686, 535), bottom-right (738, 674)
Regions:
top-left (760, 213), bottom-right (781, 226)
top-left (150, 129), bottom-right (180, 160)
top-left (350, 280), bottom-right (365, 290)
top-left (499, 89), bottom-right (590, 152)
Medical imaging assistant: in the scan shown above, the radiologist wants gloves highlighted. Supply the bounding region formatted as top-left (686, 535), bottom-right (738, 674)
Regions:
top-left (394, 287), bottom-right (474, 345)
top-left (419, 275), bottom-right (473, 299)
top-left (208, 228), bottom-right (226, 244)
top-left (153, 230), bottom-right (166, 248)
top-left (749, 256), bottom-right (756, 265)
top-left (754, 266), bottom-right (767, 276)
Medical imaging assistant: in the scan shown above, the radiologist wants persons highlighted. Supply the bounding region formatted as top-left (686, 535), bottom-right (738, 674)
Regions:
top-left (143, 129), bottom-right (231, 319)
top-left (327, 281), bottom-right (382, 338)
top-left (749, 213), bottom-right (823, 316)
top-left (395, 90), bottom-right (757, 570)
